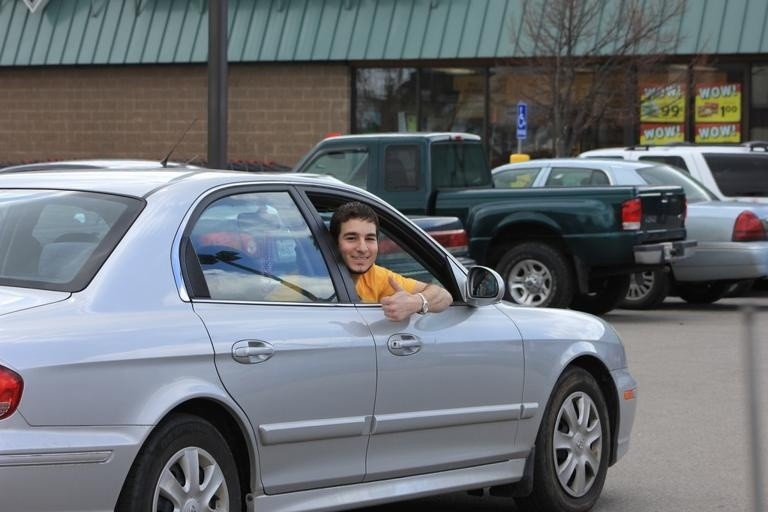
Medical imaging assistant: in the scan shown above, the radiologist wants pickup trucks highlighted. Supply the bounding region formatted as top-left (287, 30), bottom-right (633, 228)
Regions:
top-left (293, 132), bottom-right (698, 317)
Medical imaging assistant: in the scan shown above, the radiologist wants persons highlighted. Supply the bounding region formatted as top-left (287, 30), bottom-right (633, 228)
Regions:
top-left (264, 200), bottom-right (454, 320)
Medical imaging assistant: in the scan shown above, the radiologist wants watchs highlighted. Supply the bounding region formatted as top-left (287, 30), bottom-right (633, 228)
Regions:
top-left (416, 293), bottom-right (430, 316)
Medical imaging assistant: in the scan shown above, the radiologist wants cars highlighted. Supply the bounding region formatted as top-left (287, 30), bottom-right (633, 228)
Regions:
top-left (574, 139), bottom-right (768, 211)
top-left (485, 157), bottom-right (768, 308)
top-left (195, 198), bottom-right (476, 289)
top-left (0, 164), bottom-right (636, 512)
